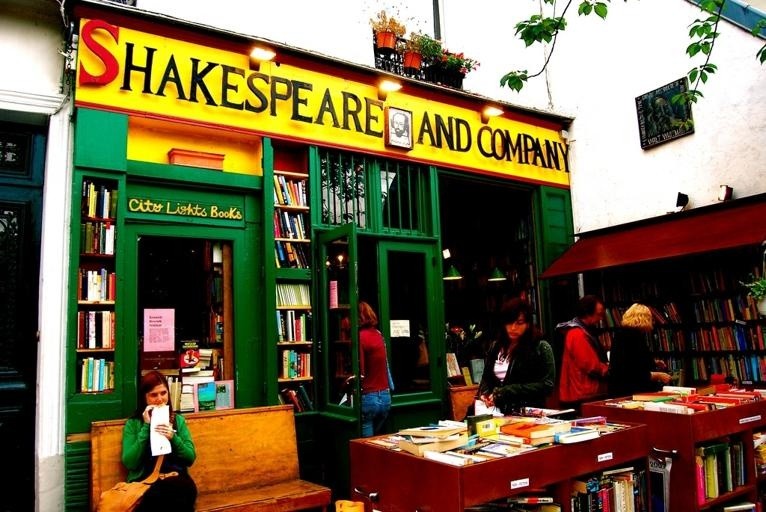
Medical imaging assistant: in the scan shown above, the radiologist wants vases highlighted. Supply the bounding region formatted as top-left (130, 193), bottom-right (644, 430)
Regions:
top-left (402, 52), bottom-right (421, 73)
top-left (427, 65), bottom-right (465, 88)
top-left (376, 32), bottom-right (396, 49)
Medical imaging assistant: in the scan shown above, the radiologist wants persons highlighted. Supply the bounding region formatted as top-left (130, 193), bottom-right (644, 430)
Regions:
top-left (390, 111), bottom-right (411, 140)
top-left (473, 297), bottom-right (558, 419)
top-left (555, 292), bottom-right (609, 418)
top-left (356, 302), bottom-right (391, 436)
top-left (118, 369), bottom-right (198, 511)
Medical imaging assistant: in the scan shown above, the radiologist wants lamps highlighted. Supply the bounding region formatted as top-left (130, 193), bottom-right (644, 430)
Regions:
top-left (481, 103), bottom-right (504, 123)
top-left (378, 79), bottom-right (403, 101)
top-left (249, 47), bottom-right (277, 71)
top-left (676, 192), bottom-right (689, 211)
top-left (718, 184), bottom-right (733, 202)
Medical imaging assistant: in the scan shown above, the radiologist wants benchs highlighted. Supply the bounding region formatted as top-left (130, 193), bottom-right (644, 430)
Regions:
top-left (90, 405), bottom-right (335, 512)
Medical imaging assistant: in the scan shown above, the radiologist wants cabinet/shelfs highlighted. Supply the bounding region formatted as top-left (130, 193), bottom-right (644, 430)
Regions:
top-left (582, 384), bottom-right (765, 512)
top-left (77, 217), bottom-right (118, 395)
top-left (350, 410), bottom-right (652, 512)
top-left (272, 169), bottom-right (319, 382)
top-left (586, 290), bottom-right (764, 385)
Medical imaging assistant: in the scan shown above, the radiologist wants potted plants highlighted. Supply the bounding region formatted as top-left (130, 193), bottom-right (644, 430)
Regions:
top-left (738, 273), bottom-right (766, 316)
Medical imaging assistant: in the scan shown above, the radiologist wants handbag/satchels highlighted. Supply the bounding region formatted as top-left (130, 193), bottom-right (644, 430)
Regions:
top-left (99, 482), bottom-right (151, 512)
top-left (386, 363), bottom-right (395, 391)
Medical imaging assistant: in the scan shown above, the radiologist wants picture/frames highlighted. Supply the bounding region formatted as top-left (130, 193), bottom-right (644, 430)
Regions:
top-left (384, 106), bottom-right (414, 151)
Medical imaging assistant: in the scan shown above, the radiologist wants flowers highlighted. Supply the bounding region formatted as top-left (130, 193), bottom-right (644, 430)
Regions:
top-left (364, 0), bottom-right (413, 36)
top-left (424, 37), bottom-right (480, 73)
top-left (402, 31), bottom-right (421, 53)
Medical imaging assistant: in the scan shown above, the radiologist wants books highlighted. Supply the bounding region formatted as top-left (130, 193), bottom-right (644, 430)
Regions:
top-left (140, 366), bottom-right (181, 376)
top-left (272, 173), bottom-right (316, 414)
top-left (165, 235), bottom-right (225, 412)
top-left (597, 263), bottom-right (766, 400)
top-left (74, 179), bottom-right (115, 392)
top-left (385, 400), bottom-right (766, 512)
